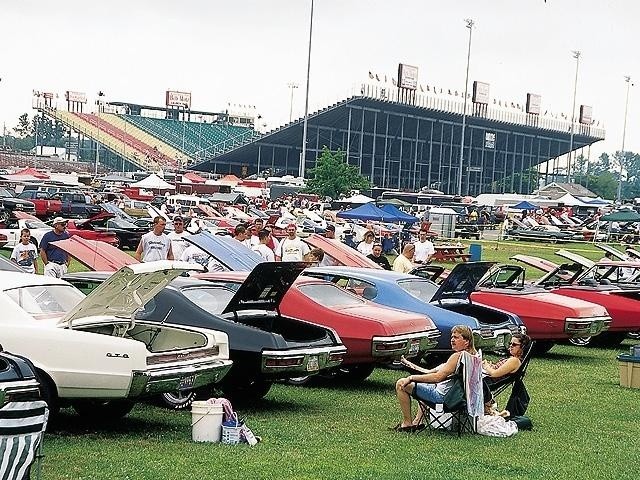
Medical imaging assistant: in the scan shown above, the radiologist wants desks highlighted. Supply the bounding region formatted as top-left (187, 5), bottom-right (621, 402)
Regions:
top-left (432, 245), bottom-right (468, 262)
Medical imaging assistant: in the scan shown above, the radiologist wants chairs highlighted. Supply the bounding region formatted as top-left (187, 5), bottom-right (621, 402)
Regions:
top-left (405, 338), bottom-right (538, 442)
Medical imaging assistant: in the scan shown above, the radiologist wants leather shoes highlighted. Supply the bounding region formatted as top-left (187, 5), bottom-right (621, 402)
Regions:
top-left (388, 425), bottom-right (424, 432)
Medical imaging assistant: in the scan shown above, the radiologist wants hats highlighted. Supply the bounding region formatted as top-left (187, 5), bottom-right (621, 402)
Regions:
top-left (53, 217), bottom-right (68, 225)
top-left (325, 225), bottom-right (335, 232)
top-left (174, 216), bottom-right (182, 222)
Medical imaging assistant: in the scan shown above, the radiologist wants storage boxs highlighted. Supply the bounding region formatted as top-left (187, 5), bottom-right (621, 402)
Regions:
top-left (618, 354), bottom-right (640, 391)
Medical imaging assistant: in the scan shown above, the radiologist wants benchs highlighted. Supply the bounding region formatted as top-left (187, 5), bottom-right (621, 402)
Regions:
top-left (431, 251), bottom-right (473, 264)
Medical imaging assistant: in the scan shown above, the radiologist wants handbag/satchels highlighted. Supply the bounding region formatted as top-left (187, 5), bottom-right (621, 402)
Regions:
top-left (503, 415), bottom-right (532, 431)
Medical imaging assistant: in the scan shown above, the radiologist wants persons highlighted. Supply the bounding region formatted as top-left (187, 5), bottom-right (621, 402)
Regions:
top-left (166, 215), bottom-right (192, 260)
top-left (460, 204), bottom-right (622, 232)
top-left (397, 331), bottom-right (533, 392)
top-left (597, 244), bottom-right (640, 282)
top-left (232, 192), bottom-right (436, 274)
top-left (8, 226), bottom-right (40, 276)
top-left (84, 179), bottom-right (228, 218)
top-left (131, 145), bottom-right (182, 167)
top-left (39, 214), bottom-right (71, 279)
top-left (135, 216), bottom-right (174, 265)
top-left (388, 323), bottom-right (480, 435)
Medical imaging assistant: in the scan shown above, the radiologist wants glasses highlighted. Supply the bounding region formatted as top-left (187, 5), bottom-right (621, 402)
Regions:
top-left (57, 222), bottom-right (66, 226)
top-left (175, 223), bottom-right (182, 225)
top-left (288, 229), bottom-right (294, 231)
top-left (374, 242), bottom-right (382, 246)
top-left (509, 341), bottom-right (520, 347)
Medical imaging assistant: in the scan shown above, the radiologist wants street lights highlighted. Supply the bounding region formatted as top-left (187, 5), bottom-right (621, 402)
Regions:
top-left (287, 82), bottom-right (299, 124)
top-left (456, 17), bottom-right (476, 195)
top-left (565, 50), bottom-right (583, 183)
top-left (617, 73), bottom-right (631, 203)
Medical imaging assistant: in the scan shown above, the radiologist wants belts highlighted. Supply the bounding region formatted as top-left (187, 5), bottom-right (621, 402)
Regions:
top-left (48, 260), bottom-right (65, 265)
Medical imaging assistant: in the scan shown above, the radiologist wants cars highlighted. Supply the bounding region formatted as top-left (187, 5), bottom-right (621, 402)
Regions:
top-left (181, 231), bottom-right (529, 369)
top-left (1, 259), bottom-right (234, 423)
top-left (0, 182), bottom-right (481, 261)
top-left (299, 234), bottom-right (614, 357)
top-left (192, 270), bottom-right (443, 388)
top-left (0, 350), bottom-right (49, 438)
top-left (58, 259), bottom-right (349, 402)
top-left (505, 203), bottom-right (639, 348)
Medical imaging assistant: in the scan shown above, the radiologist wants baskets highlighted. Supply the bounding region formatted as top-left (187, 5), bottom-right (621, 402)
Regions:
top-left (222, 412), bottom-right (240, 445)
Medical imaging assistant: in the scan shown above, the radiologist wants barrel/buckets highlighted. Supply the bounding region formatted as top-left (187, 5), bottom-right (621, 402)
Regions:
top-left (189, 400), bottom-right (226, 443)
